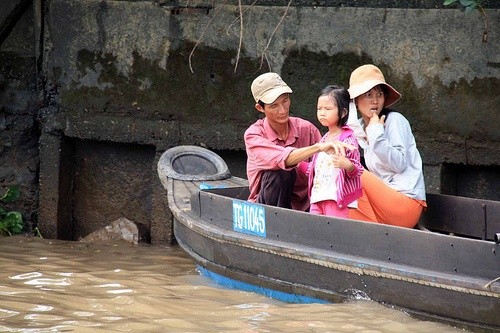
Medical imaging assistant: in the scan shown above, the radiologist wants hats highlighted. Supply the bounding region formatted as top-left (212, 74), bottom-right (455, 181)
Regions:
top-left (251, 71), bottom-right (293, 104)
top-left (347, 64), bottom-right (402, 109)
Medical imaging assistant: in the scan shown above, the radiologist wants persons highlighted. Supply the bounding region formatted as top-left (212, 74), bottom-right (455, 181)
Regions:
top-left (295, 84), bottom-right (364, 219)
top-left (345, 63), bottom-right (428, 229)
top-left (242, 72), bottom-right (358, 209)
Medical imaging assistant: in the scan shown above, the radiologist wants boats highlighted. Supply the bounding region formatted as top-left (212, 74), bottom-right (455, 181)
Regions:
top-left (157, 145), bottom-right (500, 333)
